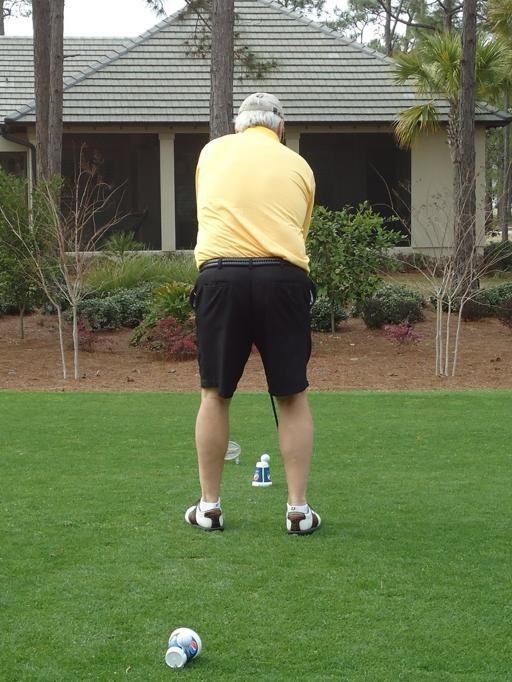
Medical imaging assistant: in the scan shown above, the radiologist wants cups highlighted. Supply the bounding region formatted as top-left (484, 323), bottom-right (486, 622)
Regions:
top-left (165, 627), bottom-right (202, 669)
top-left (251, 462), bottom-right (273, 487)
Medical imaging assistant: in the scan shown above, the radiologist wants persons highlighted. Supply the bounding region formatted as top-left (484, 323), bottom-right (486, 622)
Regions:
top-left (184, 92), bottom-right (320, 535)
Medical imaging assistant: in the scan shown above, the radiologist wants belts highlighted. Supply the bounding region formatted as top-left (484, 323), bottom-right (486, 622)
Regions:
top-left (198, 257), bottom-right (284, 268)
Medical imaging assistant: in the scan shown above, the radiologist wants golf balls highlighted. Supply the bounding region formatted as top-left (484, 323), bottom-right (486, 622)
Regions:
top-left (261, 453), bottom-right (270, 462)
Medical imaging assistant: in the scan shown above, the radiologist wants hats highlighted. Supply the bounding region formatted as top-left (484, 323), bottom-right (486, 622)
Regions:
top-left (239, 93), bottom-right (284, 119)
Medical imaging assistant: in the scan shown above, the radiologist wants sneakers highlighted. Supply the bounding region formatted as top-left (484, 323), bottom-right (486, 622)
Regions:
top-left (185, 505), bottom-right (223, 530)
top-left (285, 505), bottom-right (321, 535)
